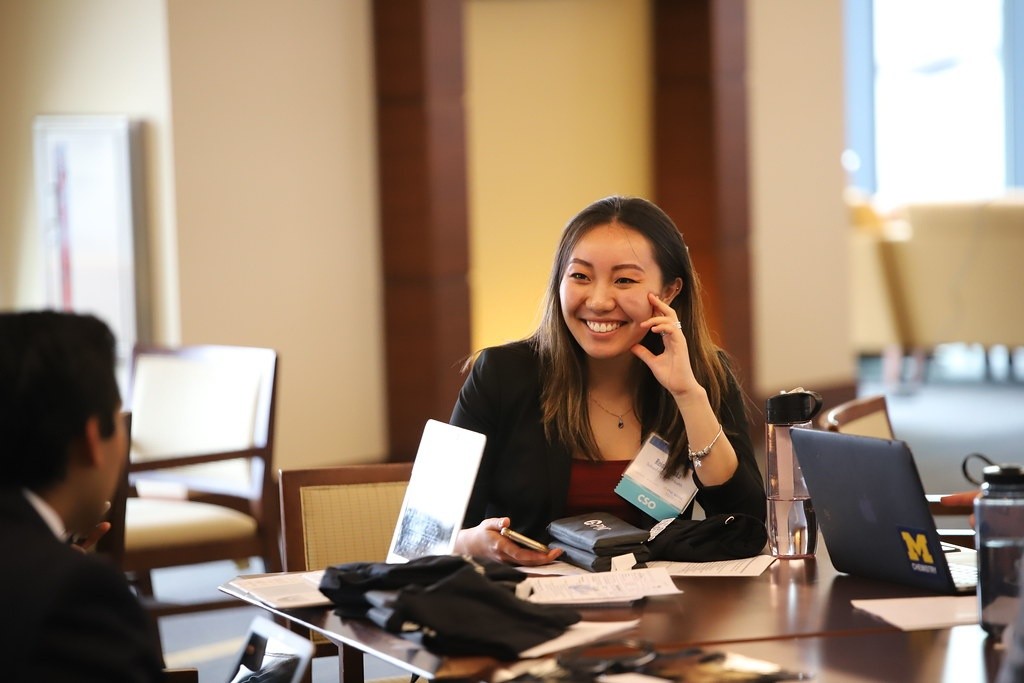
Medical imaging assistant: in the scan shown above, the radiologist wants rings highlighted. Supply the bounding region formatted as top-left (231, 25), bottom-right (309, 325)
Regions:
top-left (660, 321), bottom-right (682, 337)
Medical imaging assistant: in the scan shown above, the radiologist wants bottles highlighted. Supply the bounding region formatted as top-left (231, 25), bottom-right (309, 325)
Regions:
top-left (764, 387), bottom-right (823, 560)
top-left (961, 452), bottom-right (1024, 640)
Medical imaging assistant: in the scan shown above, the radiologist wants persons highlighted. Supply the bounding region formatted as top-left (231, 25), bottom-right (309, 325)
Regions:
top-left (0, 310), bottom-right (165, 683)
top-left (447, 195), bottom-right (768, 569)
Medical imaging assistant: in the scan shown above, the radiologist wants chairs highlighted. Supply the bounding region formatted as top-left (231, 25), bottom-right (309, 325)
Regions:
top-left (820, 396), bottom-right (980, 548)
top-left (843, 200), bottom-right (1024, 391)
top-left (89, 343), bottom-right (417, 683)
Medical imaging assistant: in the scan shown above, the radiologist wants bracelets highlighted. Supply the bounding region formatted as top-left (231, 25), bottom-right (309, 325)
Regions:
top-left (687, 424), bottom-right (723, 468)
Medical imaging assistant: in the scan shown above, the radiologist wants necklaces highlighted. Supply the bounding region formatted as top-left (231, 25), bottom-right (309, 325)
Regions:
top-left (587, 393), bottom-right (635, 429)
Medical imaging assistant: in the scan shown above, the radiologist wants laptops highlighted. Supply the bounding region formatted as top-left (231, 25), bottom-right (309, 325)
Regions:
top-left (233, 418), bottom-right (487, 609)
top-left (790, 426), bottom-right (978, 594)
top-left (227, 614), bottom-right (317, 683)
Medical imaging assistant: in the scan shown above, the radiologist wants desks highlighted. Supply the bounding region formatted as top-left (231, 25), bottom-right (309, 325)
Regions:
top-left (216, 537), bottom-right (1024, 683)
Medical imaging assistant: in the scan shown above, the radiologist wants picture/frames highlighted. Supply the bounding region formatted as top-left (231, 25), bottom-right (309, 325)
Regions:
top-left (32, 114), bottom-right (139, 411)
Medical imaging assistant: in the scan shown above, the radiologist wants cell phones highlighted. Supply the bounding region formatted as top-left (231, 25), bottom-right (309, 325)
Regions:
top-left (501, 527), bottom-right (550, 554)
